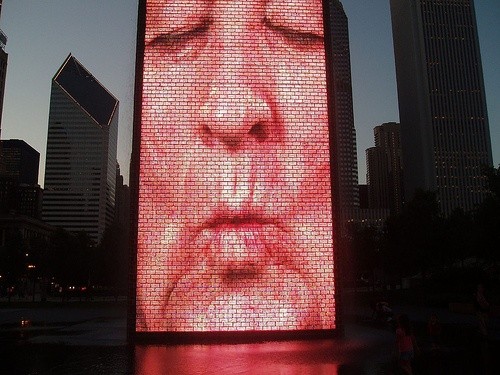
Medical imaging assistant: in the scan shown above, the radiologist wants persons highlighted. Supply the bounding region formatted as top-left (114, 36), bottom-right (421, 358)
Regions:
top-left (133, 0), bottom-right (338, 335)
top-left (393, 313), bottom-right (422, 375)
